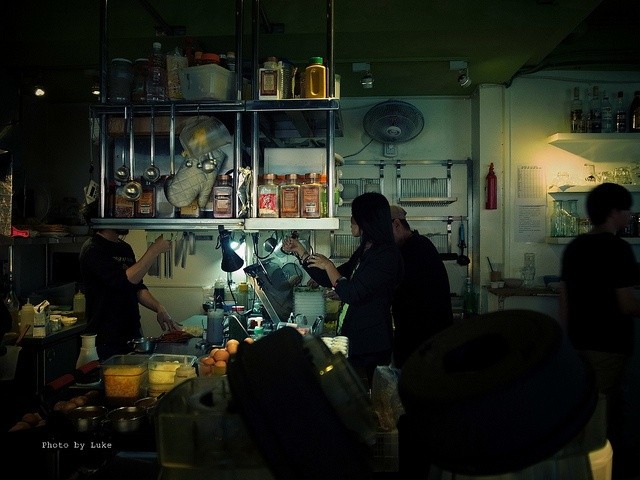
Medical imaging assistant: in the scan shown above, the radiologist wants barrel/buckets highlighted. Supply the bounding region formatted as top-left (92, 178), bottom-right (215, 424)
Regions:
top-left (304, 57), bottom-right (327, 98)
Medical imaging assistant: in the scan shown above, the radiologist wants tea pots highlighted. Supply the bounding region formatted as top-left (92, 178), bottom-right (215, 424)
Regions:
top-left (551, 200), bottom-right (572, 237)
top-left (564, 200), bottom-right (580, 237)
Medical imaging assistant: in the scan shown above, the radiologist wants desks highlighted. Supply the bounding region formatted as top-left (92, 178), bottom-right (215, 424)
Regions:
top-left (0, 329), bottom-right (238, 480)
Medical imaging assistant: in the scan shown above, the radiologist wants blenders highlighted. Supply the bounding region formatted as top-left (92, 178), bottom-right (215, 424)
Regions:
top-left (522, 253), bottom-right (536, 288)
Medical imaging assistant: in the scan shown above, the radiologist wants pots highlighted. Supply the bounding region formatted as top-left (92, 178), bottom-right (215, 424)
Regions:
top-left (127, 333), bottom-right (165, 354)
top-left (103, 406), bottom-right (146, 437)
top-left (72, 406), bottom-right (109, 437)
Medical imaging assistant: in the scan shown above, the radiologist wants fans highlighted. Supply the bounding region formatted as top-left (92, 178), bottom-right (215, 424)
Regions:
top-left (363, 100), bottom-right (424, 158)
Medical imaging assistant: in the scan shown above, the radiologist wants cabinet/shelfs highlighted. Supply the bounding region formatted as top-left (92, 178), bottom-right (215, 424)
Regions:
top-left (87, 1), bottom-right (341, 231)
top-left (2, 232), bottom-right (94, 386)
top-left (546, 132), bottom-right (639, 247)
top-left (309, 159), bottom-right (472, 278)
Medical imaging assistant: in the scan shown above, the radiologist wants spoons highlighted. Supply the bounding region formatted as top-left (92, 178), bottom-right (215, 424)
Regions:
top-left (11, 226), bottom-right (29, 238)
top-left (202, 152), bottom-right (217, 173)
top-left (122, 107), bottom-right (143, 202)
top-left (197, 157), bottom-right (203, 169)
top-left (185, 157), bottom-right (193, 167)
top-left (116, 105), bottom-right (130, 179)
top-left (143, 105), bottom-right (160, 181)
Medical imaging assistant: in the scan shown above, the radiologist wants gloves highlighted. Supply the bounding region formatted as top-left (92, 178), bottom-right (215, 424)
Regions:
top-left (166, 148), bottom-right (225, 208)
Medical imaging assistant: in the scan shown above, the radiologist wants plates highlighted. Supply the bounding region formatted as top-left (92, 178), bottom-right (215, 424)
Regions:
top-left (40, 232), bottom-right (69, 237)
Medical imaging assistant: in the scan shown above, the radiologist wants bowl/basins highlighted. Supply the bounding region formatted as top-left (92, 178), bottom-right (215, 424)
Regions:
top-left (192, 355), bottom-right (227, 378)
top-left (547, 282), bottom-right (560, 293)
top-left (221, 301), bottom-right (238, 310)
top-left (62, 317), bottom-right (77, 326)
top-left (134, 397), bottom-right (160, 414)
top-left (71, 226), bottom-right (89, 234)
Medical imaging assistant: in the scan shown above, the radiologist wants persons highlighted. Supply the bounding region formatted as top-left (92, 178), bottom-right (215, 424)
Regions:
top-left (560, 184), bottom-right (639, 339)
top-left (381, 206), bottom-right (452, 367)
top-left (79, 202), bottom-right (183, 354)
top-left (283, 192), bottom-right (396, 388)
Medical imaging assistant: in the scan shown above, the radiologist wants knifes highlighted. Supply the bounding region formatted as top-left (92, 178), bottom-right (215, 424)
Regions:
top-left (175, 232), bottom-right (186, 267)
top-left (147, 234), bottom-right (163, 279)
top-left (181, 233), bottom-right (189, 268)
top-left (190, 232), bottom-right (195, 255)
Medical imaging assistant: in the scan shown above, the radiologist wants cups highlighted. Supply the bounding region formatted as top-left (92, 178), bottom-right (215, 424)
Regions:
top-left (181, 116), bottom-right (233, 162)
top-left (613, 167), bottom-right (633, 184)
top-left (498, 281), bottom-right (504, 288)
top-left (491, 282), bottom-right (499, 289)
top-left (631, 166), bottom-right (640, 185)
top-left (599, 171), bottom-right (618, 184)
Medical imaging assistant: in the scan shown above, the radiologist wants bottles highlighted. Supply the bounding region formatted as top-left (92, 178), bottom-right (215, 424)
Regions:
top-left (239, 283), bottom-right (248, 306)
top-left (20, 298), bottom-right (34, 336)
top-left (625, 215), bottom-right (634, 235)
top-left (213, 175), bottom-right (233, 218)
top-left (219, 55), bottom-right (230, 71)
top-left (491, 263), bottom-right (501, 281)
top-left (319, 174), bottom-right (328, 218)
top-left (74, 290), bottom-right (86, 321)
top-left (258, 173), bottom-right (281, 218)
top-left (627, 91), bottom-right (640, 133)
top-left (203, 289), bottom-right (214, 311)
top-left (587, 85), bottom-right (602, 133)
top-left (259, 61), bottom-right (280, 100)
top-left (131, 58), bottom-right (149, 105)
top-left (145, 42), bottom-right (166, 102)
top-left (115, 177), bottom-right (134, 217)
top-left (230, 306), bottom-right (247, 343)
top-left (136, 176), bottom-right (156, 218)
top-left (602, 93), bottom-right (614, 133)
top-left (638, 213), bottom-right (640, 237)
top-left (298, 175), bottom-right (305, 186)
top-left (155, 175), bottom-right (176, 218)
top-left (614, 91), bottom-right (627, 133)
top-left (108, 58), bottom-right (133, 105)
top-left (227, 52), bottom-right (235, 72)
top-left (215, 288), bottom-right (224, 302)
top-left (578, 218), bottom-right (590, 235)
top-left (194, 52), bottom-right (203, 66)
top-left (301, 173), bottom-right (320, 218)
top-left (276, 176), bottom-right (285, 186)
top-left (278, 174), bottom-right (302, 218)
top-left (569, 88), bottom-right (586, 133)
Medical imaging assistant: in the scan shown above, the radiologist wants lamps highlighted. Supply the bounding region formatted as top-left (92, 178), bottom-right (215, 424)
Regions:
top-left (456, 63), bottom-right (472, 89)
top-left (218, 234), bottom-right (244, 273)
top-left (359, 67), bottom-right (374, 89)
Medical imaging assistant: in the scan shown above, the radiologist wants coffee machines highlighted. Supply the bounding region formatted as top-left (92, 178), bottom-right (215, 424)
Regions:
top-left (196, 308), bottom-right (229, 354)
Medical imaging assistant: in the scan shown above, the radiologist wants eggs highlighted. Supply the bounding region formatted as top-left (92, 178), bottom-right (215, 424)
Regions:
top-left (54, 394), bottom-right (88, 411)
top-left (197, 337), bottom-right (256, 378)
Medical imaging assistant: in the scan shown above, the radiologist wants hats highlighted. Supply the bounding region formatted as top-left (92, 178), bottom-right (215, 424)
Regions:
top-left (389, 205), bottom-right (407, 220)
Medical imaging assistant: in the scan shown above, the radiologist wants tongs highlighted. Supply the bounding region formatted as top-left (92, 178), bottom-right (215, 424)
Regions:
top-left (312, 316), bottom-right (323, 333)
top-left (164, 232), bottom-right (173, 278)
